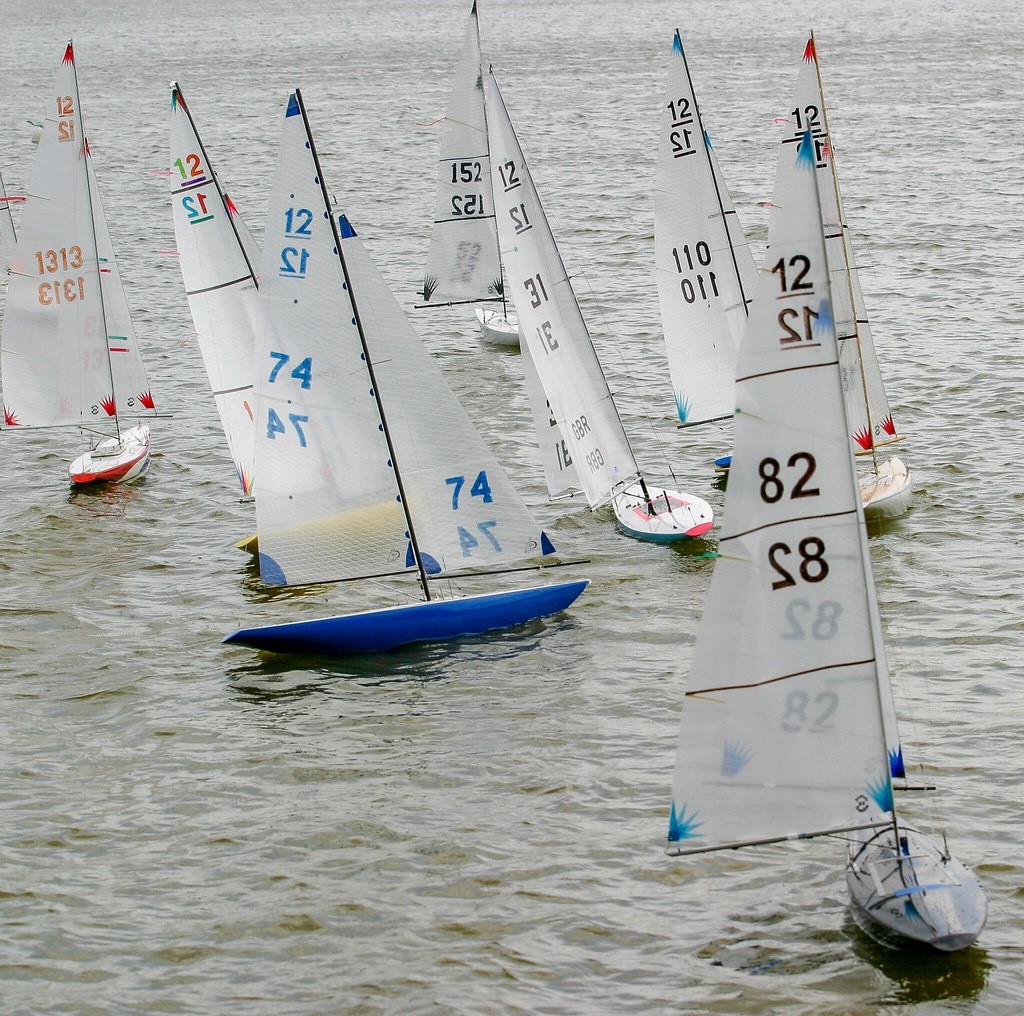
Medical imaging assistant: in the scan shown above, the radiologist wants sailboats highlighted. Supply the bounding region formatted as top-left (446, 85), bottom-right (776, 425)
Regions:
top-left (480, 51), bottom-right (716, 546)
top-left (171, 81), bottom-right (427, 563)
top-left (412, 0), bottom-right (520, 345)
top-left (221, 86), bottom-right (592, 657)
top-left (654, 28), bottom-right (759, 474)
top-left (0, 176), bottom-right (18, 296)
top-left (0, 38), bottom-right (175, 484)
top-left (661, 115), bottom-right (989, 955)
top-left (769, 26), bottom-right (914, 520)
